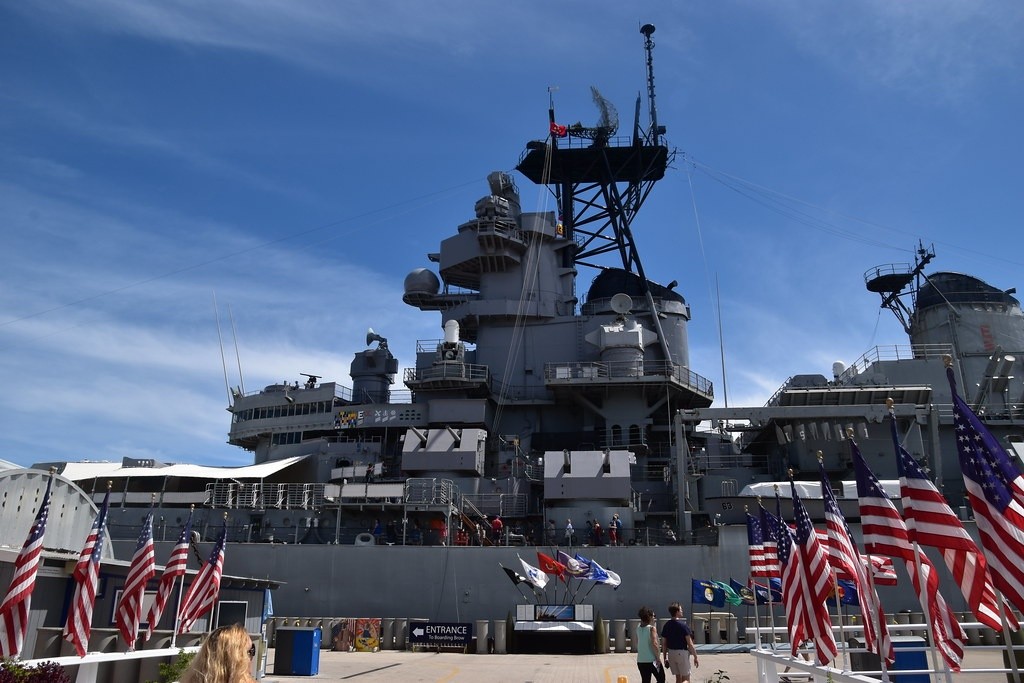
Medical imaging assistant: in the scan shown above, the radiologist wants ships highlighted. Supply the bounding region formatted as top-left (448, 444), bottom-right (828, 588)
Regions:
top-left (30, 20), bottom-right (1024, 638)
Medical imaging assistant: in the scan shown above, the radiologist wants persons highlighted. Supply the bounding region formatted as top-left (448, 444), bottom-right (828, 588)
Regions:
top-left (182, 624), bottom-right (260, 683)
top-left (371, 514), bottom-right (622, 546)
top-left (661, 603), bottom-right (698, 683)
top-left (636, 607), bottom-right (665, 683)
top-left (293, 381), bottom-right (310, 390)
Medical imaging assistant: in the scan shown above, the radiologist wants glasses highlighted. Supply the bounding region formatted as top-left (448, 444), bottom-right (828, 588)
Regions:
top-left (650, 615), bottom-right (656, 617)
top-left (246, 643), bottom-right (255, 656)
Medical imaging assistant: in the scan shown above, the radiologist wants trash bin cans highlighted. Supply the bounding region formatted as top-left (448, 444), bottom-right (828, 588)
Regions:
top-left (274, 627), bottom-right (322, 676)
top-left (847, 636), bottom-right (931, 683)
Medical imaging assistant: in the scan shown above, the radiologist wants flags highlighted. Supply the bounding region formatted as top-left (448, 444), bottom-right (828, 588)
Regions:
top-left (263, 586), bottom-right (273, 623)
top-left (550, 121), bottom-right (565, 135)
top-left (692, 578), bottom-right (755, 608)
top-left (746, 477), bottom-right (897, 665)
top-left (0, 475), bottom-right (52, 662)
top-left (519, 551), bottom-right (621, 589)
top-left (847, 435), bottom-right (968, 673)
top-left (889, 409), bottom-right (1020, 634)
top-left (144, 512), bottom-right (193, 642)
top-left (947, 369), bottom-right (1024, 615)
top-left (819, 462), bottom-right (895, 669)
top-left (502, 567), bottom-right (534, 589)
top-left (115, 508), bottom-right (155, 649)
top-left (179, 520), bottom-right (226, 635)
top-left (63, 489), bottom-right (110, 660)
